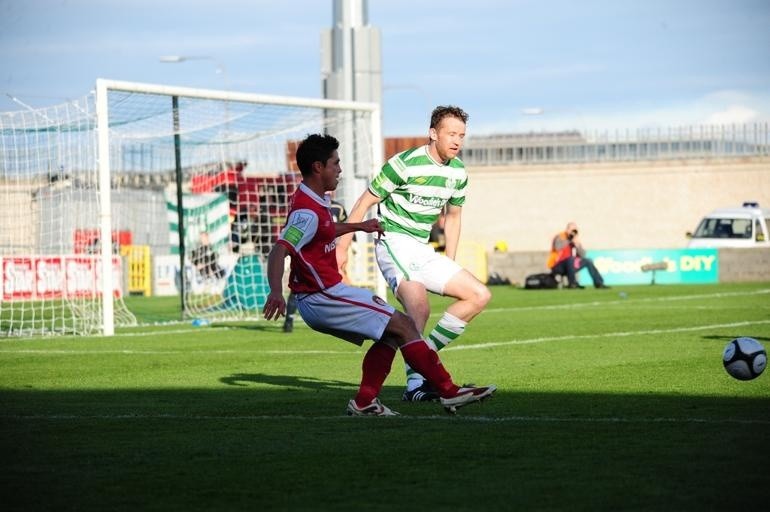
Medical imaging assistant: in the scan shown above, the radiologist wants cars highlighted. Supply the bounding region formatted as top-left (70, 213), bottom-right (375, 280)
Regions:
top-left (686, 207), bottom-right (770, 247)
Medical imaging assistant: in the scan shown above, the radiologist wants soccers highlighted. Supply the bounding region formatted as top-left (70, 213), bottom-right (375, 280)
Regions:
top-left (723, 337), bottom-right (767, 380)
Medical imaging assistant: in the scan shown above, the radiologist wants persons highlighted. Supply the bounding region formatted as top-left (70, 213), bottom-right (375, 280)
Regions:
top-left (263, 132), bottom-right (497, 416)
top-left (335, 104), bottom-right (493, 402)
top-left (548, 222), bottom-right (611, 289)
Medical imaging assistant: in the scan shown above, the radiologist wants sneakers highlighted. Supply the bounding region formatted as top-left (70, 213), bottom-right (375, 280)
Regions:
top-left (346, 396), bottom-right (402, 417)
top-left (401, 380), bottom-right (440, 402)
top-left (437, 384), bottom-right (497, 412)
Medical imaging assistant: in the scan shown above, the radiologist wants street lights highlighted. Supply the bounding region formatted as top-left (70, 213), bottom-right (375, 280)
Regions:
top-left (522, 107), bottom-right (588, 159)
top-left (160, 55), bottom-right (228, 131)
